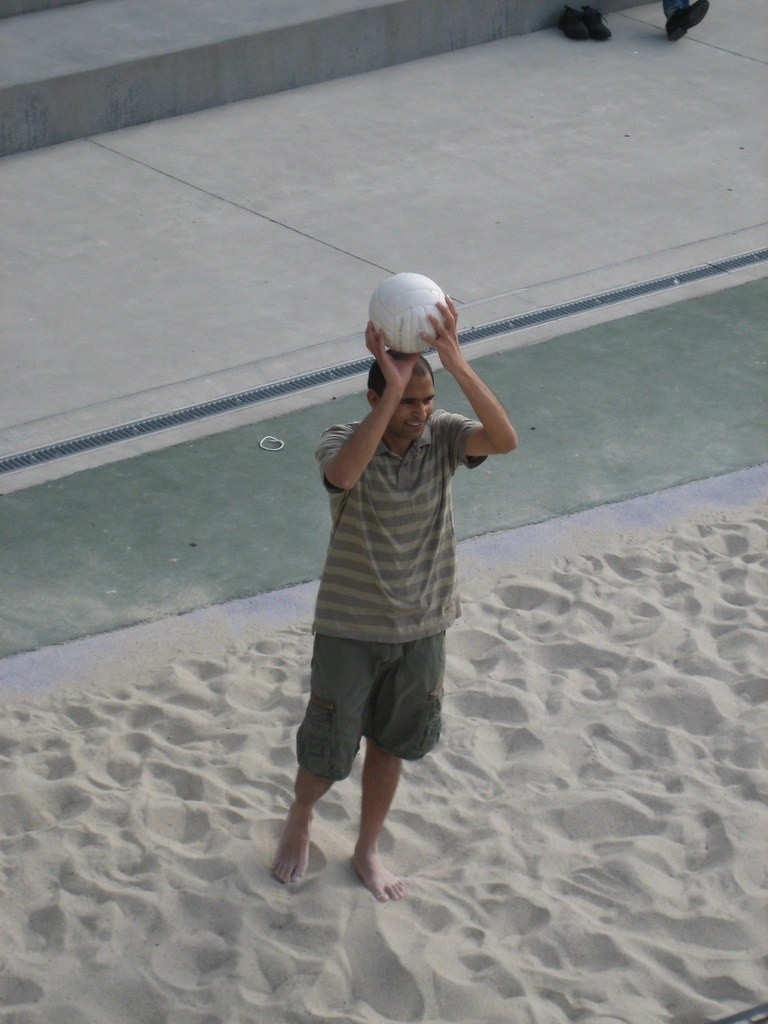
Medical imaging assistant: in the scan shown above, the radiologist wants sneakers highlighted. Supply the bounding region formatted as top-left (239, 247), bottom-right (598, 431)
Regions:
top-left (581, 6), bottom-right (612, 39)
top-left (558, 5), bottom-right (589, 39)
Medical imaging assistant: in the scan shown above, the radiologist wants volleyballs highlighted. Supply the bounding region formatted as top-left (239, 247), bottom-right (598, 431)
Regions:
top-left (369, 271), bottom-right (449, 353)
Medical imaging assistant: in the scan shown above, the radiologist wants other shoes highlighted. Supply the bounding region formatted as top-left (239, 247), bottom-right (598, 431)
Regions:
top-left (665, 0), bottom-right (709, 41)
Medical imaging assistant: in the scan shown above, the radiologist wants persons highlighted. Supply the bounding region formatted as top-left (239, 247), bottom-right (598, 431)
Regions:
top-left (271, 295), bottom-right (518, 905)
top-left (662, 0), bottom-right (710, 43)
